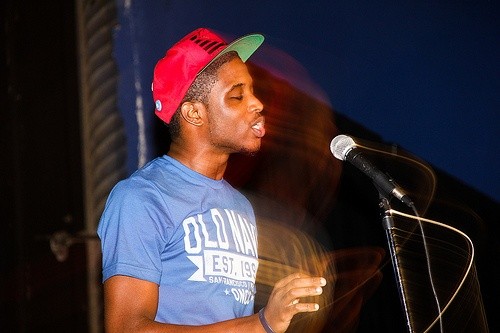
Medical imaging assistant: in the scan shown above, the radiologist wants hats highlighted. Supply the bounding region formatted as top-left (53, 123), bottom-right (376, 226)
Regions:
top-left (151, 27), bottom-right (264, 124)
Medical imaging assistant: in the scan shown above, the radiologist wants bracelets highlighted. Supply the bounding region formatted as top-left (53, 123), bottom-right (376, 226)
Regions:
top-left (259, 306), bottom-right (273, 333)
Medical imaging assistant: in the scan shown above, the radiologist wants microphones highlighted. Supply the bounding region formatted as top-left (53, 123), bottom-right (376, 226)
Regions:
top-left (329, 135), bottom-right (414, 207)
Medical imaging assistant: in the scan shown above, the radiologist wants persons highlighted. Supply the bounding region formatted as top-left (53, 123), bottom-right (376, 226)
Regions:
top-left (97, 28), bottom-right (327, 333)
